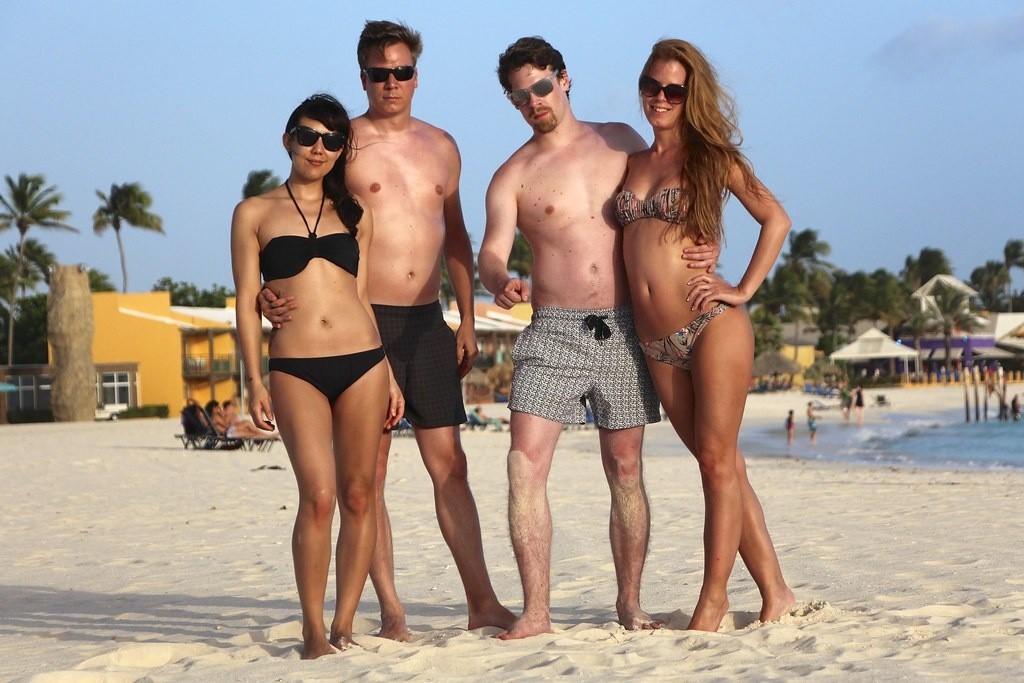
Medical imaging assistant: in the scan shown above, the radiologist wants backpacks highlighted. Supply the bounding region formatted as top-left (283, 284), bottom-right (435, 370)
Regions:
top-left (181, 402), bottom-right (209, 437)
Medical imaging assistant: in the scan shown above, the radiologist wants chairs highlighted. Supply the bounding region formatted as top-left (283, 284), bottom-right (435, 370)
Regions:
top-left (174, 397), bottom-right (281, 452)
top-left (813, 400), bottom-right (840, 413)
top-left (801, 383), bottom-right (839, 397)
top-left (469, 412), bottom-right (502, 432)
top-left (873, 394), bottom-right (891, 409)
top-left (394, 418), bottom-right (414, 438)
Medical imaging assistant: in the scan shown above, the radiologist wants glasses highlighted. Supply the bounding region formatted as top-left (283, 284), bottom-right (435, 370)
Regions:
top-left (288, 125), bottom-right (346, 152)
top-left (637, 75), bottom-right (688, 104)
top-left (505, 68), bottom-right (559, 107)
top-left (362, 65), bottom-right (416, 83)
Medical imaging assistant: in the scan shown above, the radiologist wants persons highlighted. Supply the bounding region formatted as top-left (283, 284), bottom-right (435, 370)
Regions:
top-left (256, 20), bottom-right (520, 643)
top-left (461, 405), bottom-right (510, 432)
top-left (477, 34), bottom-right (722, 641)
top-left (808, 402), bottom-right (819, 445)
top-left (614, 39), bottom-right (793, 632)
top-left (206, 400), bottom-right (281, 440)
top-left (786, 410), bottom-right (795, 446)
top-left (1012, 394), bottom-right (1021, 421)
top-left (231, 93), bottom-right (406, 659)
top-left (837, 371), bottom-right (864, 427)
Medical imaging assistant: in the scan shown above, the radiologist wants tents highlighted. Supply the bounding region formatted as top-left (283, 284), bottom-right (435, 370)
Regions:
top-left (831, 326), bottom-right (923, 388)
